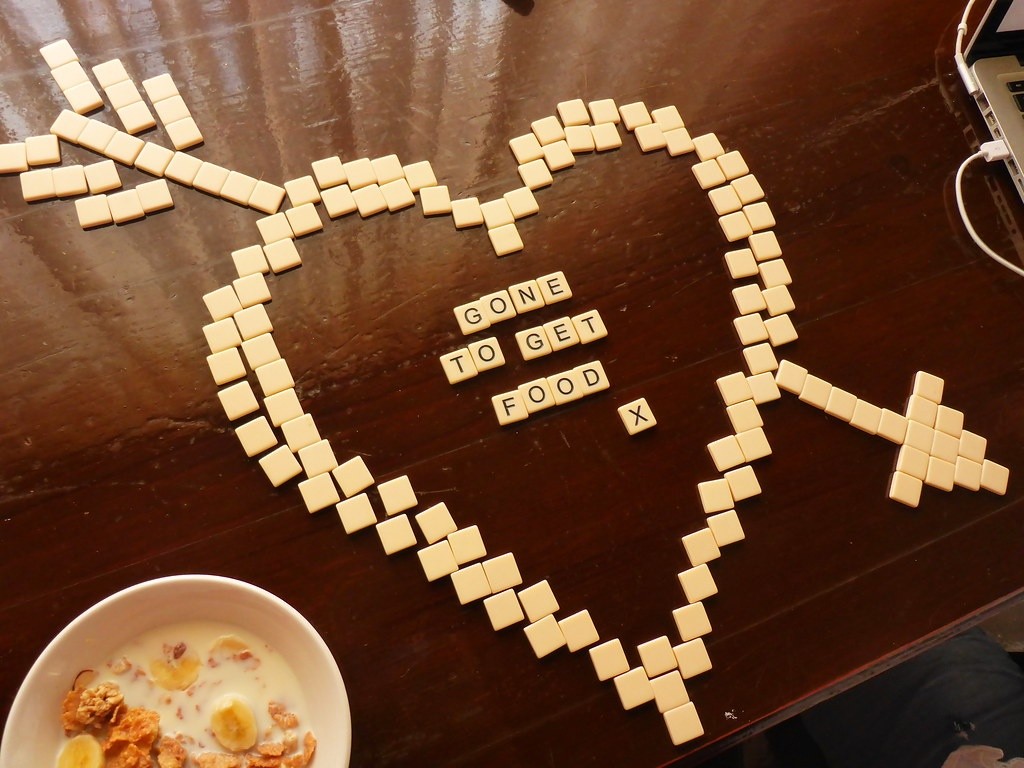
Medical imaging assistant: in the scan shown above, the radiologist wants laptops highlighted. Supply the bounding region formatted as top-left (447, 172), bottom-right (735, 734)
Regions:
top-left (959, 0), bottom-right (1024, 205)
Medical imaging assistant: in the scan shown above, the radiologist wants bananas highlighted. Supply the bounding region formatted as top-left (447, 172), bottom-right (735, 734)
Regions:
top-left (150, 649), bottom-right (202, 692)
top-left (55, 734), bottom-right (106, 768)
top-left (209, 700), bottom-right (257, 752)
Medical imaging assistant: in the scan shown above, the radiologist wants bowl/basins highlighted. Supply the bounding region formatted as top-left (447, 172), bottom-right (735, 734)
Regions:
top-left (0, 574), bottom-right (352, 768)
